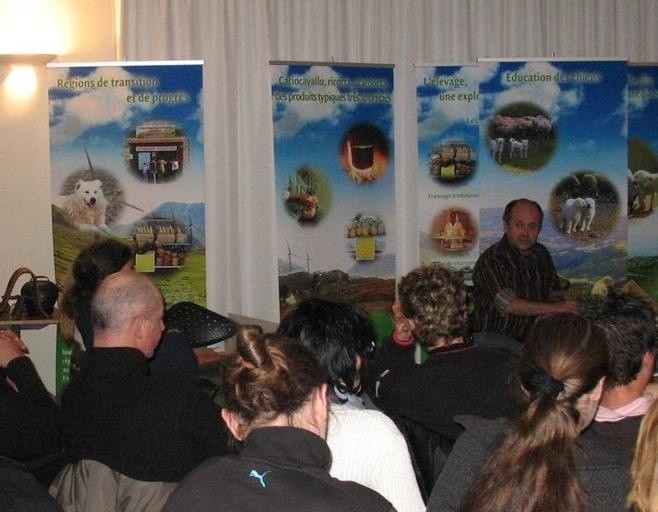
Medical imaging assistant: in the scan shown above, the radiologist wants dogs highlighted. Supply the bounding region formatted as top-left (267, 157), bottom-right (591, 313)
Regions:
top-left (559, 197), bottom-right (596, 235)
top-left (490, 137), bottom-right (504, 164)
top-left (632, 170), bottom-right (658, 211)
top-left (508, 138), bottom-right (528, 160)
top-left (60, 179), bottom-right (112, 237)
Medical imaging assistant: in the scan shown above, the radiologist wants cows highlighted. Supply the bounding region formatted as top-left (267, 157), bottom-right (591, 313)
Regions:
top-left (304, 269), bottom-right (353, 298)
top-left (590, 276), bottom-right (614, 301)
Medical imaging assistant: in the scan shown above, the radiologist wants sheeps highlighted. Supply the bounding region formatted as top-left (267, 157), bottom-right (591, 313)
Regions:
top-left (493, 114), bottom-right (553, 140)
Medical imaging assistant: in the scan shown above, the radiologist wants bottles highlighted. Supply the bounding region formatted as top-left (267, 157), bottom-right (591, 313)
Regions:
top-left (21, 280), bottom-right (59, 316)
